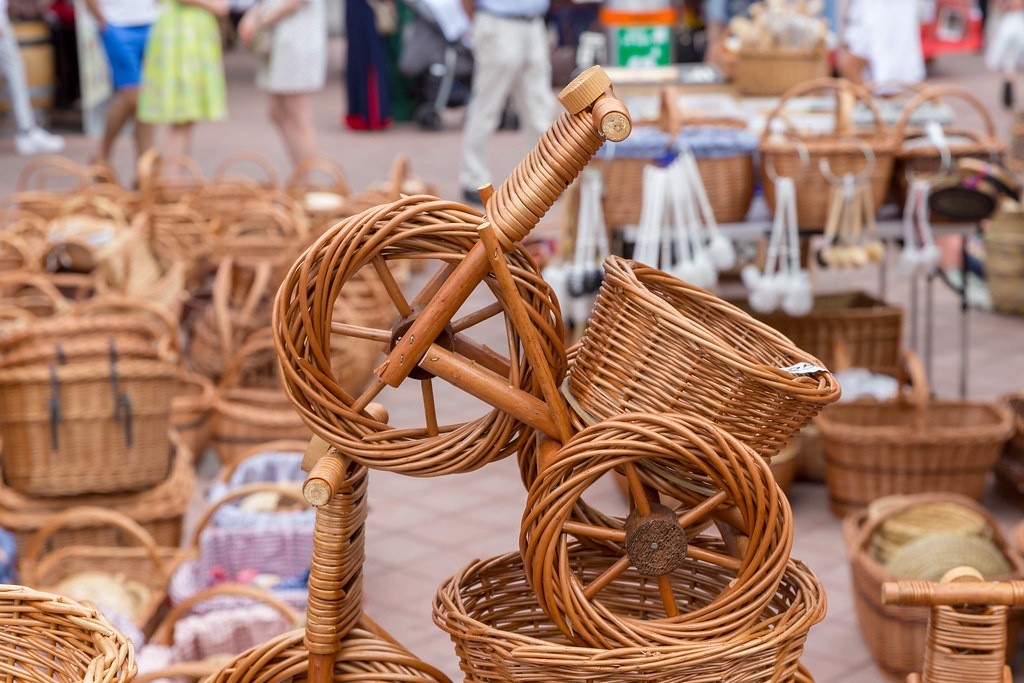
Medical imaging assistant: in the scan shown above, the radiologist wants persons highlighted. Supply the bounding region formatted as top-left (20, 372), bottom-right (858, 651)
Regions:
top-left (344, 0), bottom-right (397, 130)
top-left (239, 0), bottom-right (329, 170)
top-left (457, 0), bottom-right (559, 202)
top-left (83, 0), bottom-right (159, 183)
top-left (137, 0), bottom-right (230, 176)
top-left (0, 0), bottom-right (64, 156)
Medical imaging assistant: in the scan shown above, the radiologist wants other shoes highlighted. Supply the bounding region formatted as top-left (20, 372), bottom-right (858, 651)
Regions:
top-left (15, 128), bottom-right (66, 155)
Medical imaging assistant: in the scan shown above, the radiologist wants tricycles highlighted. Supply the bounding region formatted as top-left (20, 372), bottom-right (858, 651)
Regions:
top-left (272, 65), bottom-right (840, 654)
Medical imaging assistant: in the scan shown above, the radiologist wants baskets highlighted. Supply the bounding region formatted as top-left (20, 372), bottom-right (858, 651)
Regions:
top-left (4, 15), bottom-right (1024, 678)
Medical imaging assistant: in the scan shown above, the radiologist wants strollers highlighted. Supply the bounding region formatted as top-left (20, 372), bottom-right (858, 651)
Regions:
top-left (398, 0), bottom-right (519, 131)
